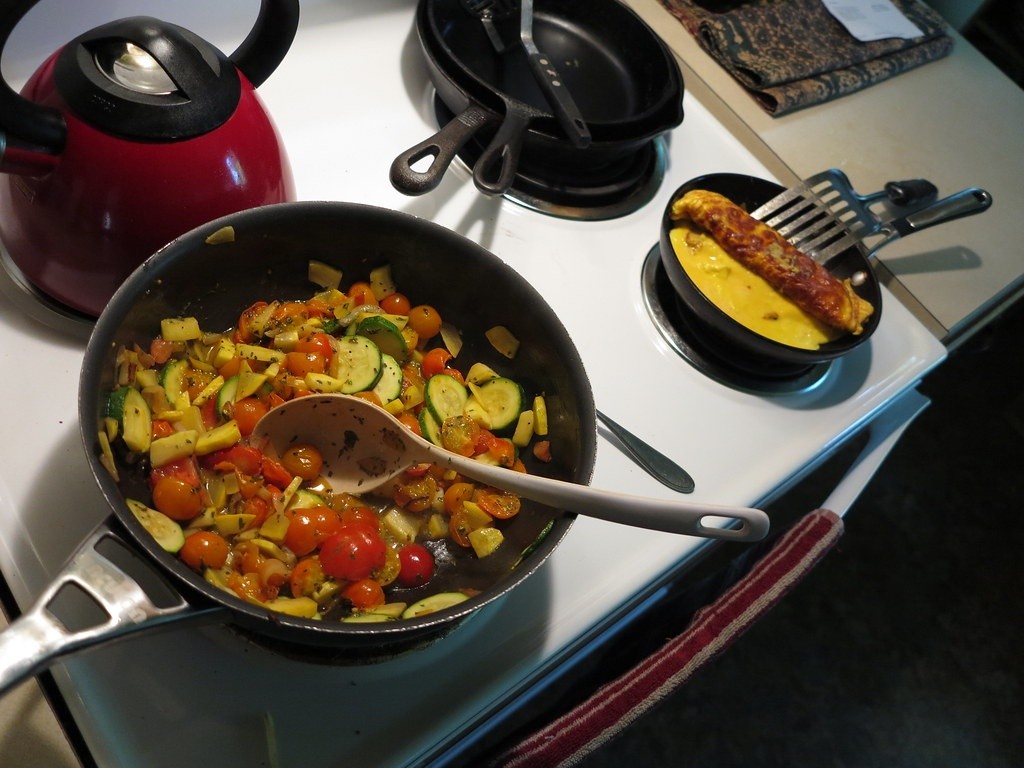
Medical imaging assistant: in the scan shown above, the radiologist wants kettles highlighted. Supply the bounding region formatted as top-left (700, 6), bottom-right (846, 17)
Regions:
top-left (0, 0), bottom-right (301, 323)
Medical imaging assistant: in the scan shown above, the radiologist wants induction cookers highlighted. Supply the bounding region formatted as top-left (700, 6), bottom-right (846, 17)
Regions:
top-left (0, 0), bottom-right (952, 767)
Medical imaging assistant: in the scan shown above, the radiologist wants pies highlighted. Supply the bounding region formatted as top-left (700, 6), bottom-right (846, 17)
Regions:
top-left (668, 187), bottom-right (874, 351)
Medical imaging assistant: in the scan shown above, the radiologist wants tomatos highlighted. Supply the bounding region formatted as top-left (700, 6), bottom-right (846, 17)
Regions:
top-left (97, 260), bottom-right (552, 624)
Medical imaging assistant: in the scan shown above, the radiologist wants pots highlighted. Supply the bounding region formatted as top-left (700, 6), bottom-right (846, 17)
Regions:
top-left (426, 1), bottom-right (680, 195)
top-left (659, 169), bottom-right (992, 364)
top-left (0, 200), bottom-right (597, 710)
top-left (387, 1), bottom-right (684, 196)
